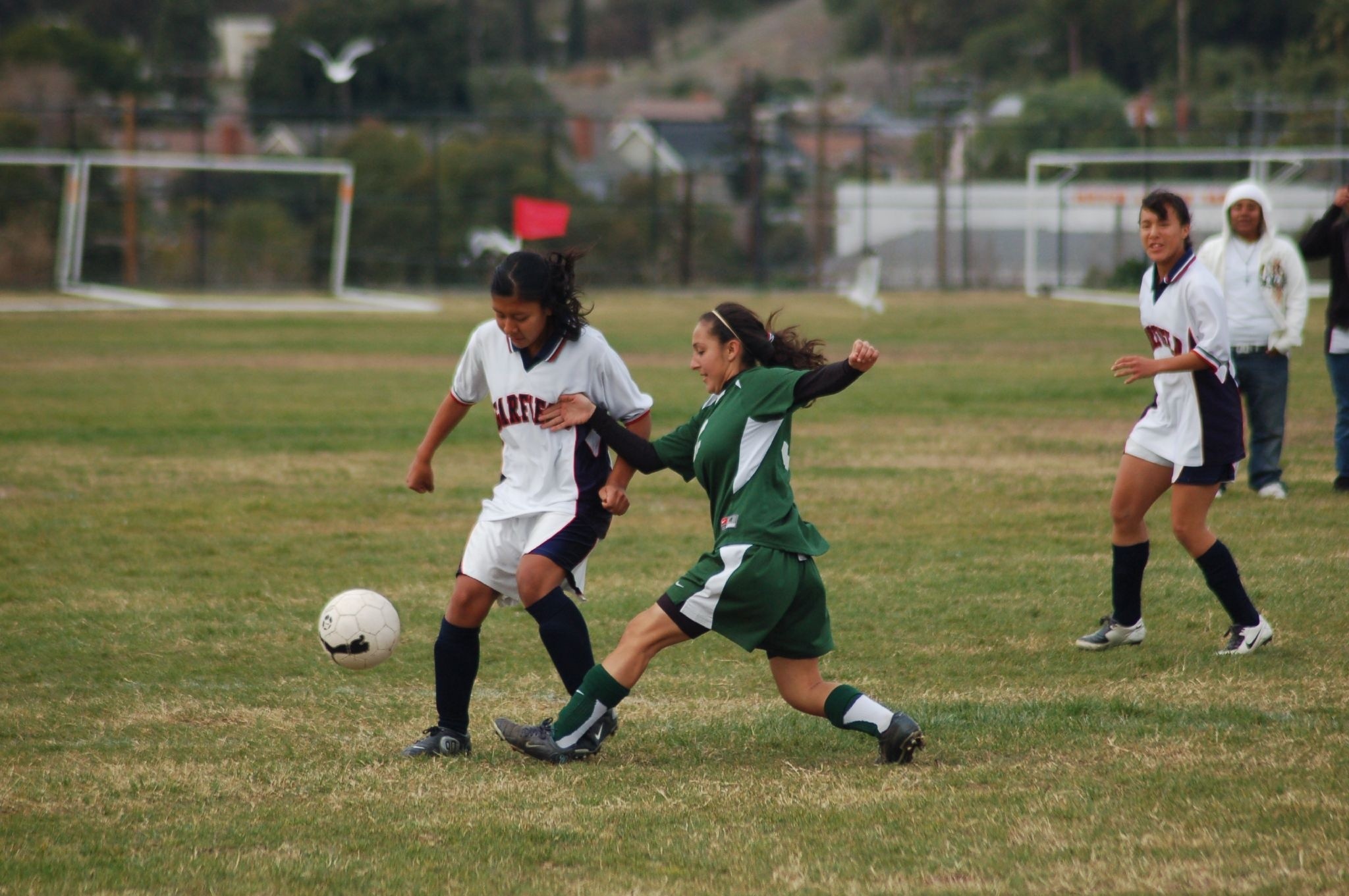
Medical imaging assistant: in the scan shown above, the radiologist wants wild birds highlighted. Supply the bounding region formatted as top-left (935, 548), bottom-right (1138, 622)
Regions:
top-left (295, 34), bottom-right (388, 84)
top-left (832, 252), bottom-right (888, 317)
top-left (465, 222), bottom-right (525, 259)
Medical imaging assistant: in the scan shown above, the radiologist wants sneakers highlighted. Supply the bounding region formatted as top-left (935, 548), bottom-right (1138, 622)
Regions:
top-left (494, 717), bottom-right (576, 767)
top-left (405, 726), bottom-right (471, 759)
top-left (1074, 614), bottom-right (1147, 651)
top-left (873, 712), bottom-right (925, 767)
top-left (1214, 614), bottom-right (1272, 656)
top-left (575, 707), bottom-right (619, 759)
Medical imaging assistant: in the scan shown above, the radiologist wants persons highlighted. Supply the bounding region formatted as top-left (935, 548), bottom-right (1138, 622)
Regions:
top-left (1199, 174), bottom-right (1311, 502)
top-left (402, 252), bottom-right (655, 755)
top-left (1074, 188), bottom-right (1273, 651)
top-left (1300, 186), bottom-right (1349, 490)
top-left (490, 301), bottom-right (928, 768)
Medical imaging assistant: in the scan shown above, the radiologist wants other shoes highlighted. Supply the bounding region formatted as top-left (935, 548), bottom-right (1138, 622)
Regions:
top-left (1215, 488), bottom-right (1223, 498)
top-left (1333, 475), bottom-right (1349, 491)
top-left (1257, 483), bottom-right (1285, 500)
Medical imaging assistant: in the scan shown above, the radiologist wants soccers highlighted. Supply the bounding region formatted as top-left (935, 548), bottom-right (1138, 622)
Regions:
top-left (320, 591), bottom-right (399, 667)
